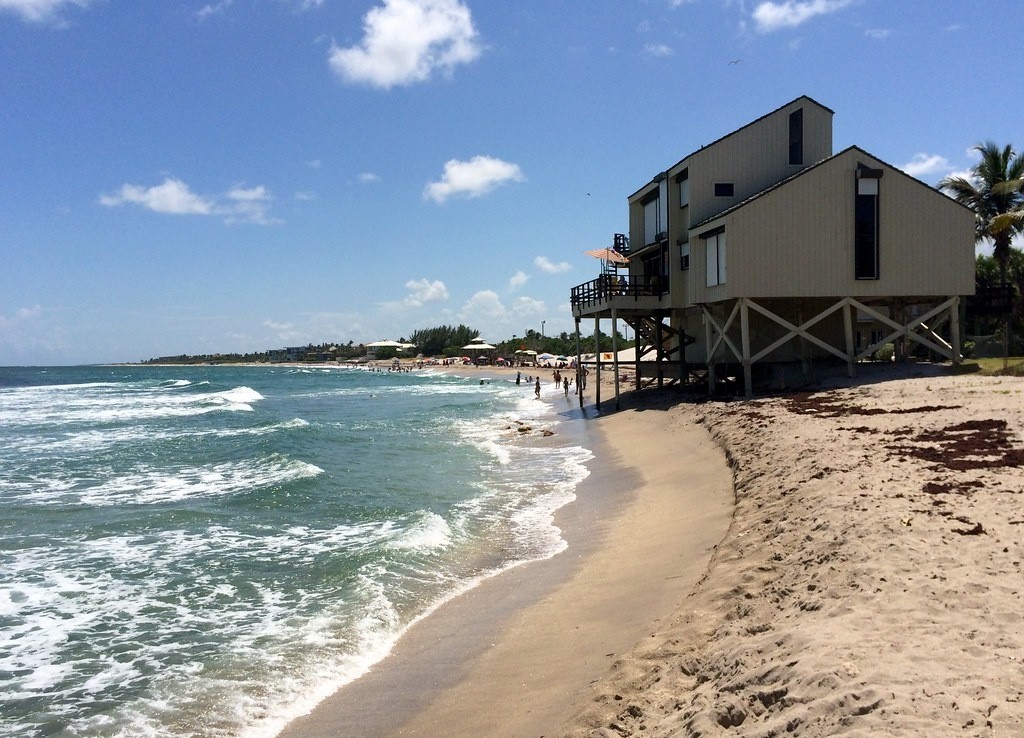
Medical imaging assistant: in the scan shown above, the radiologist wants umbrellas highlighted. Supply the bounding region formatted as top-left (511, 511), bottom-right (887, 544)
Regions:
top-left (585, 248), bottom-right (630, 274)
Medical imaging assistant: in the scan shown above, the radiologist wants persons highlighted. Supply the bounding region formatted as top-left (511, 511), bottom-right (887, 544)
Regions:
top-left (347, 358), bottom-right (606, 399)
top-left (596, 274), bottom-right (627, 294)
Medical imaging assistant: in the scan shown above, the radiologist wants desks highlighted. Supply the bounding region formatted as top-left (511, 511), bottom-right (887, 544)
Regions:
top-left (598, 284), bottom-right (620, 296)
